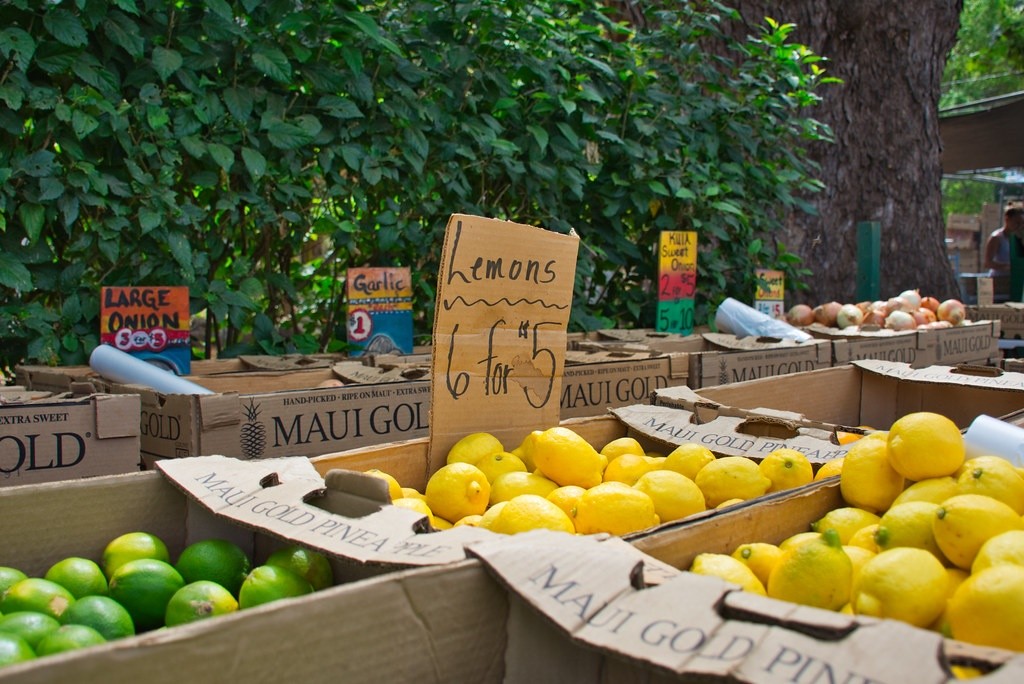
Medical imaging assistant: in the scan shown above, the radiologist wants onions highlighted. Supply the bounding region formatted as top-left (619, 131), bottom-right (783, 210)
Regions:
top-left (775, 289), bottom-right (965, 331)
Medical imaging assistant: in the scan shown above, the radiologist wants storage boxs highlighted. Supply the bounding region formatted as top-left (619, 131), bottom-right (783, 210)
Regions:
top-left (570, 335), bottom-right (832, 390)
top-left (370, 349), bottom-right (690, 420)
top-left (650, 358), bottom-right (1024, 435)
top-left (585, 326), bottom-right (709, 345)
top-left (978, 304), bottom-right (1024, 340)
top-left (89, 362), bottom-right (432, 457)
top-left (0, 381), bottom-right (142, 487)
top-left (804, 322), bottom-right (940, 370)
top-left (14, 355), bottom-right (302, 392)
top-left (600, 480), bottom-right (1024, 684)
top-left (310, 403), bottom-right (858, 684)
top-left (0, 452), bottom-right (510, 684)
top-left (919, 319), bottom-right (1003, 366)
top-left (957, 276), bottom-right (994, 305)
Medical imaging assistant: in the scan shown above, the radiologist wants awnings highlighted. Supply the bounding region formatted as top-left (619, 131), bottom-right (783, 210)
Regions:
top-left (937, 90), bottom-right (1024, 188)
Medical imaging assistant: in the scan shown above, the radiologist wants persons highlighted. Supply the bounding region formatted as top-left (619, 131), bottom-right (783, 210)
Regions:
top-left (985, 202), bottom-right (1024, 299)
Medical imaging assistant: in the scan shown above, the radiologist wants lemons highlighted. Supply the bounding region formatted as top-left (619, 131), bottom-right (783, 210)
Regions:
top-left (349, 425), bottom-right (842, 537)
top-left (688, 410), bottom-right (1024, 680)
top-left (0, 530), bottom-right (333, 669)
